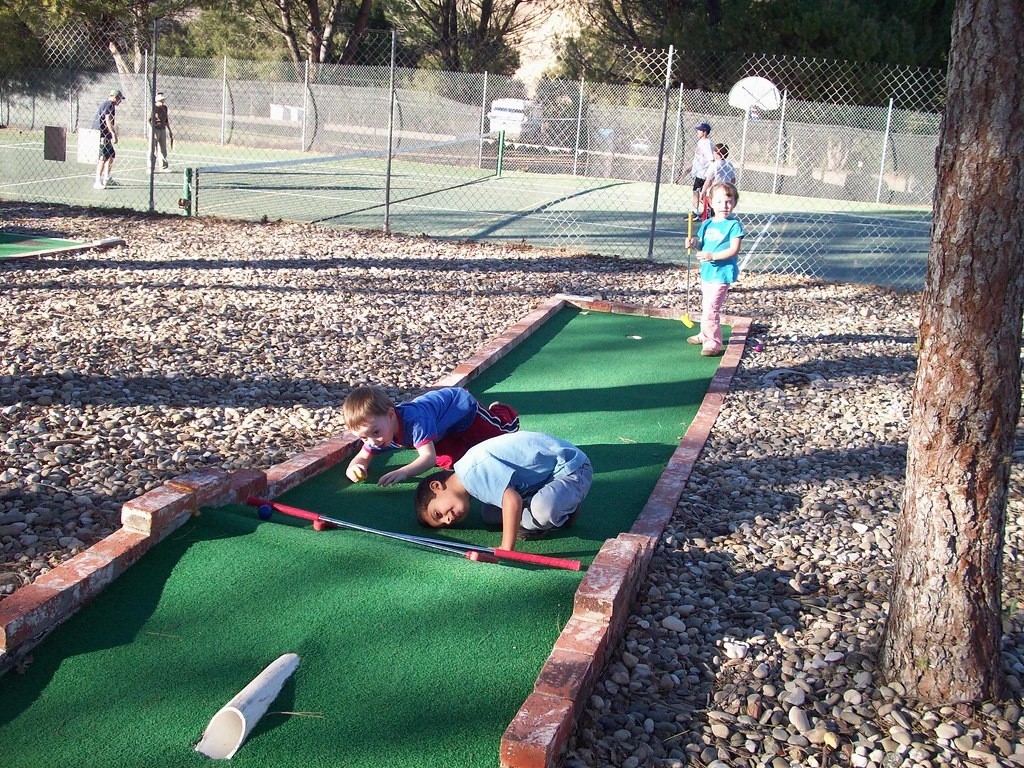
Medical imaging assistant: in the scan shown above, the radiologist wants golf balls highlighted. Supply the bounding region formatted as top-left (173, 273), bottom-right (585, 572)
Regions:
top-left (258, 504), bottom-right (272, 519)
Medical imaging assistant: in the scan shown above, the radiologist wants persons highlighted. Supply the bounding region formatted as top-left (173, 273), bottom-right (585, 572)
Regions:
top-left (414, 431), bottom-right (593, 551)
top-left (683, 122), bottom-right (736, 222)
top-left (147, 92), bottom-right (174, 173)
top-left (92, 89), bottom-right (126, 190)
top-left (343, 386), bottom-right (520, 487)
top-left (685, 181), bottom-right (745, 356)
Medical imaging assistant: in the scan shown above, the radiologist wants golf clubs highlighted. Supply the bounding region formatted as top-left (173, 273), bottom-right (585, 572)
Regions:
top-left (681, 213), bottom-right (694, 328)
top-left (246, 494), bottom-right (582, 571)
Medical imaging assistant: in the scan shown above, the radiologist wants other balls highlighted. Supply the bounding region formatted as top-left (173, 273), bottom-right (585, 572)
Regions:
top-left (755, 345), bottom-right (763, 352)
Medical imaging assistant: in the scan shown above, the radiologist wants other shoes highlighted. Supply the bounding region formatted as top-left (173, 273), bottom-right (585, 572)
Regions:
top-left (566, 503), bottom-right (581, 524)
top-left (516, 527), bottom-right (550, 541)
top-left (701, 346), bottom-right (720, 356)
top-left (687, 335), bottom-right (702, 344)
top-left (93, 181), bottom-right (106, 190)
top-left (489, 401), bottom-right (519, 418)
top-left (161, 165), bottom-right (167, 169)
top-left (684, 211), bottom-right (699, 221)
top-left (104, 177), bottom-right (120, 187)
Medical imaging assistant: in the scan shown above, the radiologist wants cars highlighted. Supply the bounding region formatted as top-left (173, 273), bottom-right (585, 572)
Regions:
top-left (629, 138), bottom-right (654, 157)
top-left (596, 128), bottom-right (617, 142)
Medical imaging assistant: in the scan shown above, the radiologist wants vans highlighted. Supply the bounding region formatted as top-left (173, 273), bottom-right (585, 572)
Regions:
top-left (487, 97), bottom-right (543, 145)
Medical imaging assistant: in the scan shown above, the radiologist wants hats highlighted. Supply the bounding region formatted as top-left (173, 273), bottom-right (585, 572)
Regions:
top-left (156, 94), bottom-right (167, 101)
top-left (696, 123), bottom-right (710, 133)
top-left (110, 90), bottom-right (126, 99)
